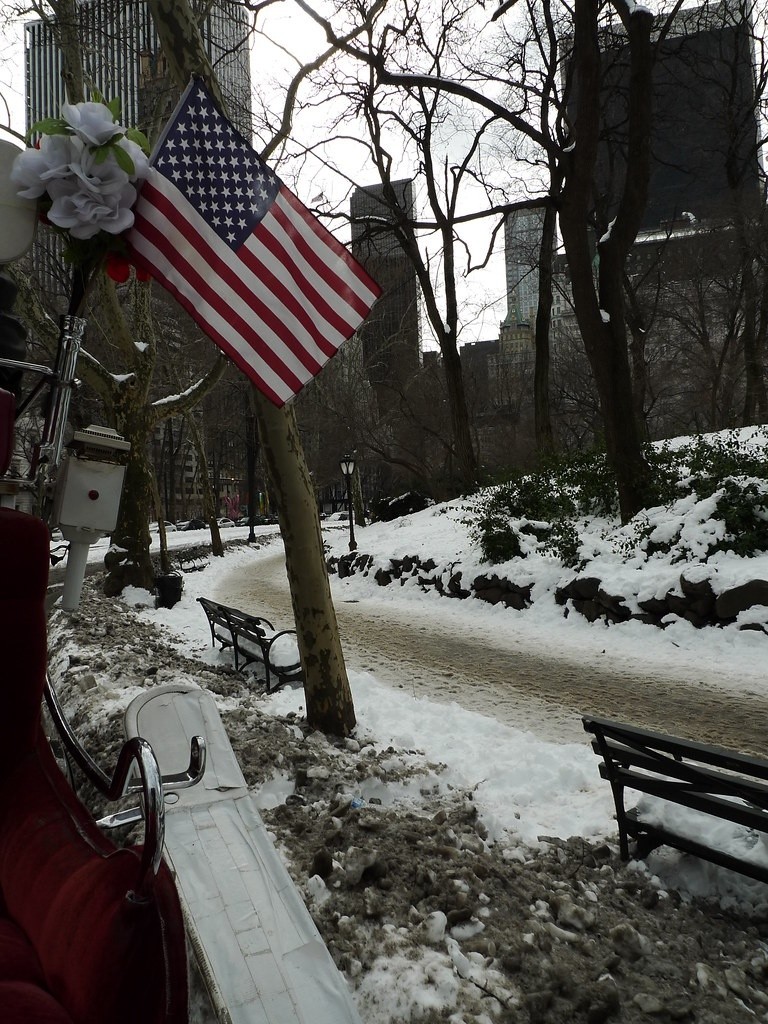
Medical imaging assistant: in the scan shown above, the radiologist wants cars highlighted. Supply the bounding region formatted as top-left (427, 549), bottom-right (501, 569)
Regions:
top-left (175, 519), bottom-right (206, 530)
top-left (261, 515), bottom-right (278, 525)
top-left (235, 516), bottom-right (265, 527)
top-left (52, 527), bottom-right (64, 542)
top-left (149, 520), bottom-right (177, 533)
top-left (205, 517), bottom-right (236, 528)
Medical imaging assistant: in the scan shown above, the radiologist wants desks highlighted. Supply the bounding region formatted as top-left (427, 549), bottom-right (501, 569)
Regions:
top-left (582, 714), bottom-right (768, 884)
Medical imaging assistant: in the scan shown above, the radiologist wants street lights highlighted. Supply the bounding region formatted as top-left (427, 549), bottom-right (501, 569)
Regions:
top-left (340, 453), bottom-right (357, 551)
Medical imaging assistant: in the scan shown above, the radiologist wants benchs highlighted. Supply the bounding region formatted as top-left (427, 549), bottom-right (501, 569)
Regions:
top-left (175, 549), bottom-right (211, 573)
top-left (197, 597), bottom-right (304, 694)
top-left (0, 385), bottom-right (206, 1024)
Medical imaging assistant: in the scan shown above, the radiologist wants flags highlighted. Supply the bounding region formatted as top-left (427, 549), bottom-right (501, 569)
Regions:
top-left (118, 76), bottom-right (383, 410)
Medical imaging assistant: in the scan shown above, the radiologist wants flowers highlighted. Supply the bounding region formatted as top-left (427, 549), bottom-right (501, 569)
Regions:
top-left (11, 88), bottom-right (154, 282)
